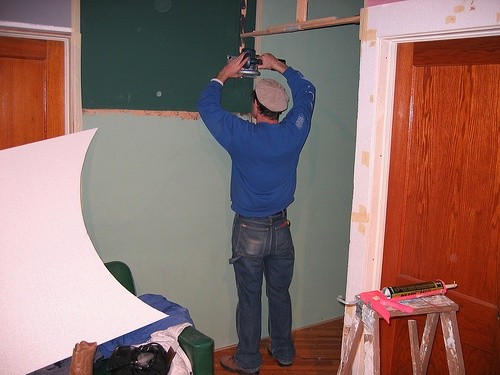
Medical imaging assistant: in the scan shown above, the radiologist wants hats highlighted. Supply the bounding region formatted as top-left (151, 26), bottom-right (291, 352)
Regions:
top-left (256, 78), bottom-right (290, 113)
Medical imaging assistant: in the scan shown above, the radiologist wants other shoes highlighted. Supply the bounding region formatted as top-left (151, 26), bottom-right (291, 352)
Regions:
top-left (220, 355), bottom-right (260, 375)
top-left (267, 344), bottom-right (293, 366)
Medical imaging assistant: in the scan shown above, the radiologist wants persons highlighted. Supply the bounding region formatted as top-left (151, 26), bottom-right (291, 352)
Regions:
top-left (198, 52), bottom-right (316, 375)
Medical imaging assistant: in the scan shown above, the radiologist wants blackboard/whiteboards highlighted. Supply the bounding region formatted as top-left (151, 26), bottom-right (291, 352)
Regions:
top-left (71, 0), bottom-right (262, 120)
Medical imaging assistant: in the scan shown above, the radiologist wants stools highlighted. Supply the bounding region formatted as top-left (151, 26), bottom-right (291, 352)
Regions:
top-left (337, 290), bottom-right (466, 375)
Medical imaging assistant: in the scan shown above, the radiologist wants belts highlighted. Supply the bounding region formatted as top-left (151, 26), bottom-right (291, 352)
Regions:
top-left (272, 209), bottom-right (286, 216)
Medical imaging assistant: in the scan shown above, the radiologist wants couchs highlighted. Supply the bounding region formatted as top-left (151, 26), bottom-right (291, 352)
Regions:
top-left (103, 261), bottom-right (214, 375)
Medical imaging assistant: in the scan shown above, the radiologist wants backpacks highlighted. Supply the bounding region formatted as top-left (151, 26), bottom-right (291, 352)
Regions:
top-left (93, 343), bottom-right (171, 375)
